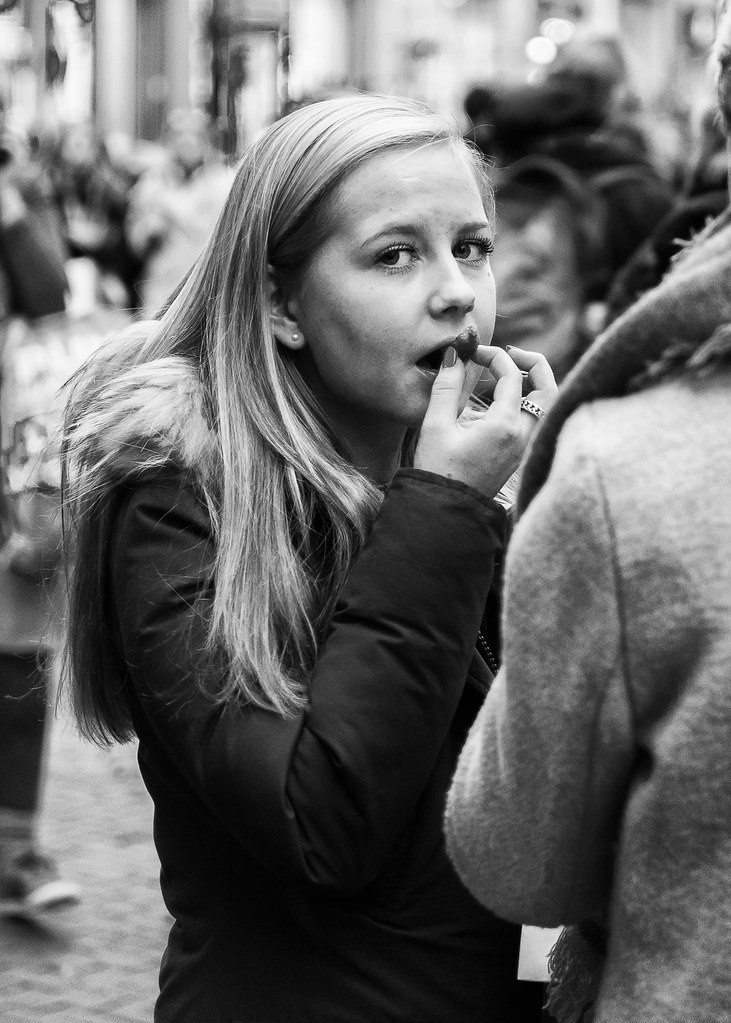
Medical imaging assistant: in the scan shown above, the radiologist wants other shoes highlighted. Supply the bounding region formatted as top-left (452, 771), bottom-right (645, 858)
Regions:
top-left (0, 848), bottom-right (81, 916)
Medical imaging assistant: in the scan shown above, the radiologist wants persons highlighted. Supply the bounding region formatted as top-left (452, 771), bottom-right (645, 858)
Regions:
top-left (0, 0), bottom-right (731, 1023)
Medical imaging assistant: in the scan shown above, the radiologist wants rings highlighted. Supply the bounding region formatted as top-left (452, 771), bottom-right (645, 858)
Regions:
top-left (521, 399), bottom-right (546, 420)
